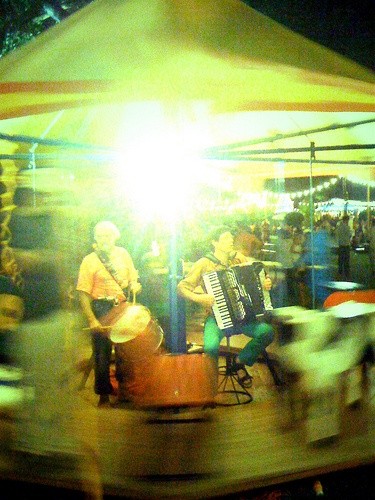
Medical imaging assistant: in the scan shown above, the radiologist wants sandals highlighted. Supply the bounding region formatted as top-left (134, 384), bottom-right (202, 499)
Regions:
top-left (233, 357), bottom-right (253, 388)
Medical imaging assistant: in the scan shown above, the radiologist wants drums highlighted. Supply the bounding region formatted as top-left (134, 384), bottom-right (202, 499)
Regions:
top-left (106, 304), bottom-right (164, 363)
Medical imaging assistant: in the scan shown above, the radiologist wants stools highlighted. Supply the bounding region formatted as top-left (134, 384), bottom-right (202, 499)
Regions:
top-left (210, 330), bottom-right (252, 407)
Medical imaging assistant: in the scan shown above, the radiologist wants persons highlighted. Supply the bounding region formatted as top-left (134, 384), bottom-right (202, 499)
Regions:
top-left (177, 227), bottom-right (275, 387)
top-left (8, 187), bottom-right (52, 248)
top-left (0, 274), bottom-right (36, 402)
top-left (233, 211), bottom-right (375, 344)
top-left (76, 221), bottom-right (142, 404)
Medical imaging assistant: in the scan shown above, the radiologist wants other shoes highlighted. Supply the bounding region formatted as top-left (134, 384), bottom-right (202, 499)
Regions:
top-left (118, 388), bottom-right (128, 402)
top-left (97, 395), bottom-right (110, 409)
